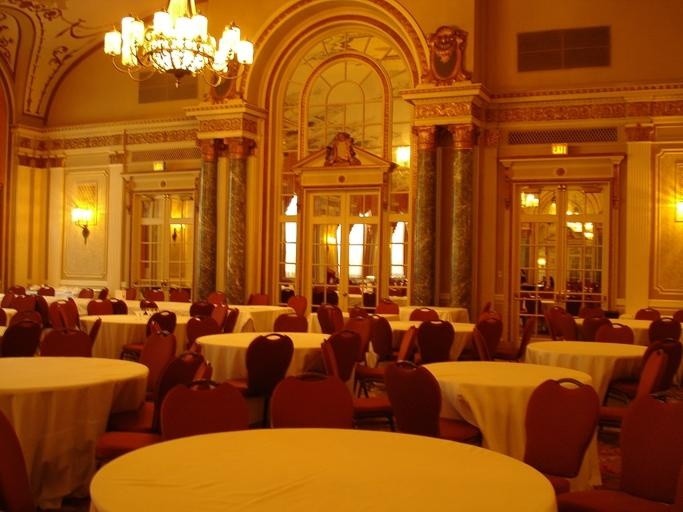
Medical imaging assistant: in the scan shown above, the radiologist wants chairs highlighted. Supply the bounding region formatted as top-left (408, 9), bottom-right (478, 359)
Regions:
top-left (161, 379), bottom-right (250, 440)
top-left (557, 389), bottom-right (683, 512)
top-left (523, 378), bottom-right (601, 494)
top-left (384, 361), bottom-right (482, 449)
top-left (94, 351), bottom-right (213, 470)
top-left (0, 411), bottom-right (33, 512)
top-left (269, 372), bottom-right (353, 430)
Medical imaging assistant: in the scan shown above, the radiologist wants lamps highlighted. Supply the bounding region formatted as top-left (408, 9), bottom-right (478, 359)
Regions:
top-left (72, 207), bottom-right (90, 247)
top-left (104, 0), bottom-right (253, 89)
